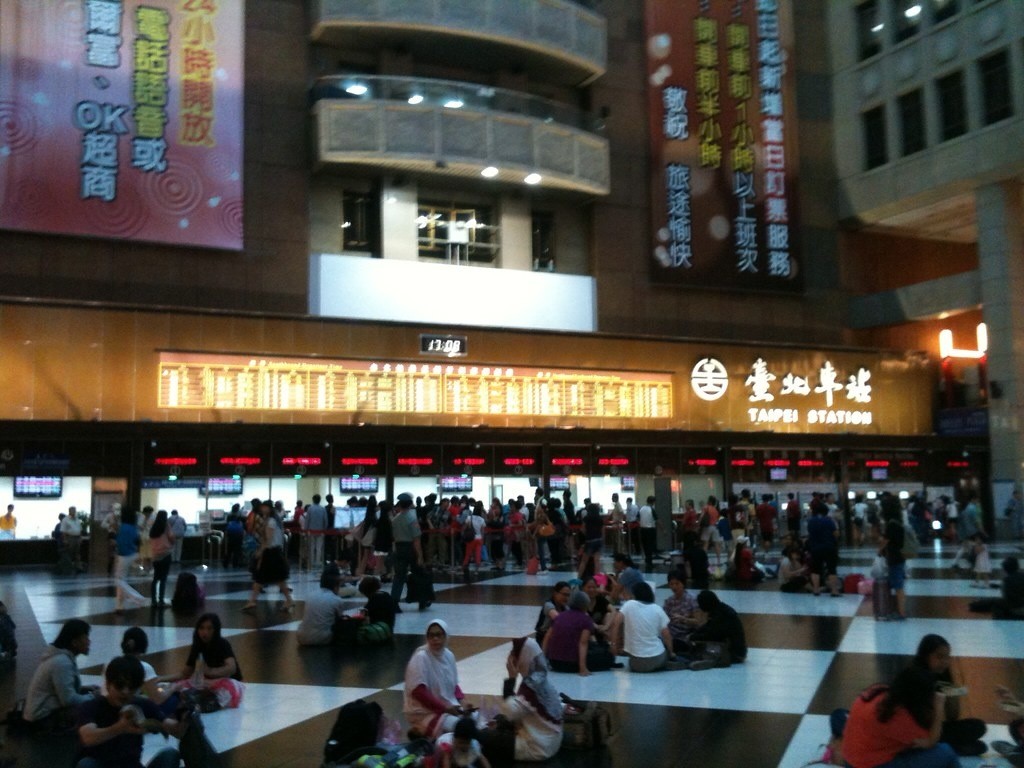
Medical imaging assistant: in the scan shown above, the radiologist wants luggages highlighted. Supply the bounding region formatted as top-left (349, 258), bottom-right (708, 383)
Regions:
top-left (576, 556), bottom-right (595, 582)
top-left (873, 554), bottom-right (893, 621)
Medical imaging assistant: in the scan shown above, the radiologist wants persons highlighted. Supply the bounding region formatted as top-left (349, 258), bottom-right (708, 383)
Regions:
top-left (0, 531), bottom-right (1024, 768)
top-left (0, 488), bottom-right (1023, 619)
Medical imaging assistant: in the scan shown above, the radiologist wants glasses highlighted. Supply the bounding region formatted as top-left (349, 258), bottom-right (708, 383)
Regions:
top-left (426, 631), bottom-right (446, 638)
top-left (110, 681), bottom-right (138, 694)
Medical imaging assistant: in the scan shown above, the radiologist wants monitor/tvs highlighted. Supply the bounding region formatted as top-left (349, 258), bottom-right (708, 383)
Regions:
top-left (538, 475), bottom-right (569, 491)
top-left (770, 468), bottom-right (787, 480)
top-left (871, 466), bottom-right (889, 481)
top-left (339, 478), bottom-right (378, 493)
top-left (200, 477), bottom-right (243, 495)
top-left (621, 477), bottom-right (635, 490)
top-left (438, 475), bottom-right (473, 492)
top-left (13, 477), bottom-right (62, 497)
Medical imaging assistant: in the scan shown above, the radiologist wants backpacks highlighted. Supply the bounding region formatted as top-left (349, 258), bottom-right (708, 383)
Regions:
top-left (324, 700), bottom-right (382, 764)
top-left (171, 572), bottom-right (205, 616)
top-left (887, 518), bottom-right (920, 558)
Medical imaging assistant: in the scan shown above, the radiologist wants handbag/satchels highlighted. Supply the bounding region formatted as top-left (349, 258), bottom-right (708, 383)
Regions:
top-left (377, 711), bottom-right (402, 744)
top-left (698, 505), bottom-right (710, 528)
top-left (331, 614), bottom-right (360, 657)
top-left (539, 513), bottom-right (556, 536)
top-left (690, 641), bottom-right (731, 668)
top-left (405, 564), bottom-right (436, 602)
top-left (843, 573), bottom-right (874, 595)
top-left (464, 515), bottom-right (475, 543)
top-left (561, 701), bottom-right (613, 752)
top-left (870, 557), bottom-right (889, 580)
top-left (526, 556), bottom-right (538, 574)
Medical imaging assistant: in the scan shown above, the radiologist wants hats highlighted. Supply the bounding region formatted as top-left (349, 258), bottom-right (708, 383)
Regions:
top-left (736, 534), bottom-right (750, 545)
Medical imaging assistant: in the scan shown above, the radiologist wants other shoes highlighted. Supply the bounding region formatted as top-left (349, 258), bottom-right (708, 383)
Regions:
top-left (661, 659), bottom-right (712, 671)
